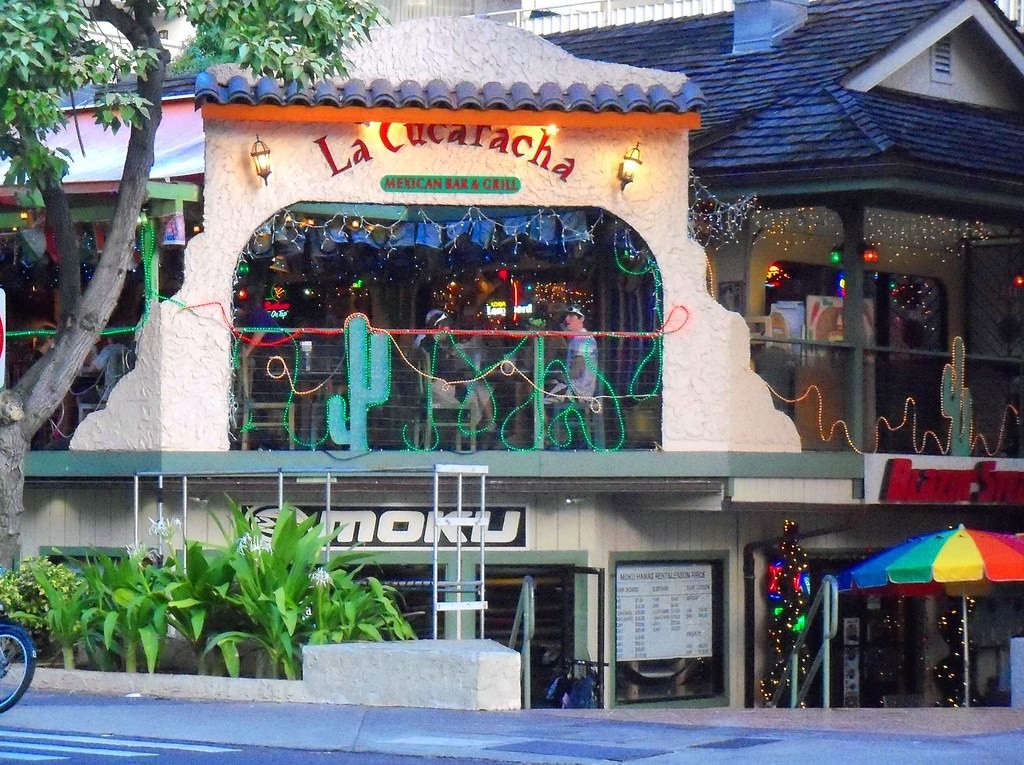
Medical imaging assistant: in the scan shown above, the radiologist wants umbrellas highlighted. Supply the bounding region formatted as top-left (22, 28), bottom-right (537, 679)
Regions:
top-left (837, 523), bottom-right (1024, 707)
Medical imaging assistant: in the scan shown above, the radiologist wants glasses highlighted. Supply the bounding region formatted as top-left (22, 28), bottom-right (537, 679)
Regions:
top-left (433, 313), bottom-right (448, 326)
top-left (565, 307), bottom-right (583, 316)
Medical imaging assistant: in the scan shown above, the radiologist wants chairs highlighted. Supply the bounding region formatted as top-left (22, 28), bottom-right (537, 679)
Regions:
top-left (408, 346), bottom-right (477, 450)
top-left (79, 350), bottom-right (135, 425)
top-left (551, 343), bottom-right (606, 450)
top-left (240, 342), bottom-right (295, 450)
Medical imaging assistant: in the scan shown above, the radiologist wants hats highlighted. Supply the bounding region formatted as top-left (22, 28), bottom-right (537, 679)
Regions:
top-left (567, 304), bottom-right (585, 317)
top-left (425, 309), bottom-right (444, 324)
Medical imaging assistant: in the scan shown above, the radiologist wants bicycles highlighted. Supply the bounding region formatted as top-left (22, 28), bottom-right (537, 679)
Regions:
top-left (0, 600), bottom-right (39, 716)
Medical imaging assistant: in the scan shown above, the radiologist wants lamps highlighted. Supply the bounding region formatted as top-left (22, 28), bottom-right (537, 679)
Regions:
top-left (251, 133), bottom-right (272, 187)
top-left (620, 141), bottom-right (643, 192)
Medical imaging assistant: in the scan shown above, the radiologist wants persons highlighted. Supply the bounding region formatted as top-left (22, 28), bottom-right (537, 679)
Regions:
top-left (75, 336), bottom-right (129, 407)
top-left (414, 310), bottom-right (507, 450)
top-left (544, 304), bottom-right (597, 446)
top-left (236, 295), bottom-right (303, 451)
top-left (25, 325), bottom-right (56, 369)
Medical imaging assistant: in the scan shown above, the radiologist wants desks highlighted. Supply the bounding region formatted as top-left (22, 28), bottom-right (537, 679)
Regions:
top-left (70, 376), bottom-right (105, 438)
top-left (437, 370), bottom-right (564, 449)
top-left (264, 371), bottom-right (408, 451)
